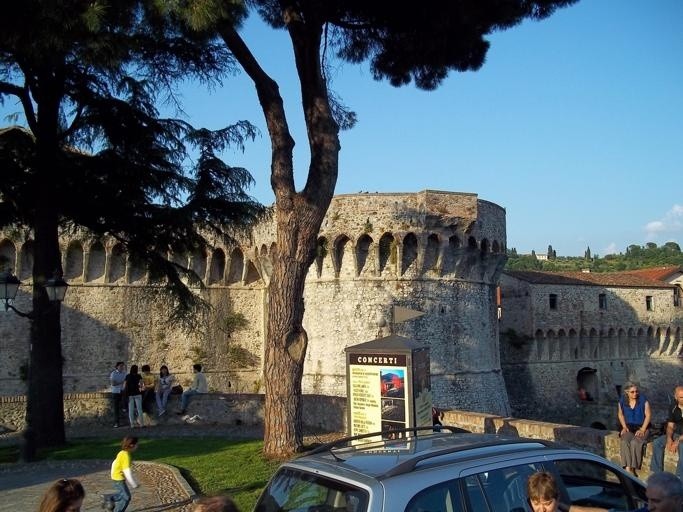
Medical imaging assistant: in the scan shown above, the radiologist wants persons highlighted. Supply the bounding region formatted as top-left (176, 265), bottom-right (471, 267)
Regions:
top-left (527, 471), bottom-right (609, 512)
top-left (617, 384), bottom-right (653, 475)
top-left (636, 470), bottom-right (683, 512)
top-left (141, 363), bottom-right (156, 416)
top-left (122, 366), bottom-right (146, 428)
top-left (100, 435), bottom-right (141, 512)
top-left (174, 363), bottom-right (208, 415)
top-left (649, 386), bottom-right (683, 479)
top-left (111, 362), bottom-right (128, 428)
top-left (187, 495), bottom-right (241, 512)
top-left (153, 365), bottom-right (174, 416)
top-left (578, 385), bottom-right (588, 401)
top-left (39, 478), bottom-right (85, 512)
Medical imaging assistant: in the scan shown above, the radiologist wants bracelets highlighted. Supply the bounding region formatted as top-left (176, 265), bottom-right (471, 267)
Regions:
top-left (639, 427), bottom-right (645, 433)
top-left (676, 436), bottom-right (679, 442)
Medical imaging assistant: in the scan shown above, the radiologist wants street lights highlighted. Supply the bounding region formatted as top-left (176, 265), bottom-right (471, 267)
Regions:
top-left (0, 269), bottom-right (69, 464)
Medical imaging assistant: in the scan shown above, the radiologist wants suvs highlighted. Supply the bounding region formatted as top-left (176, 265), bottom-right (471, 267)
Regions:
top-left (248, 424), bottom-right (651, 512)
